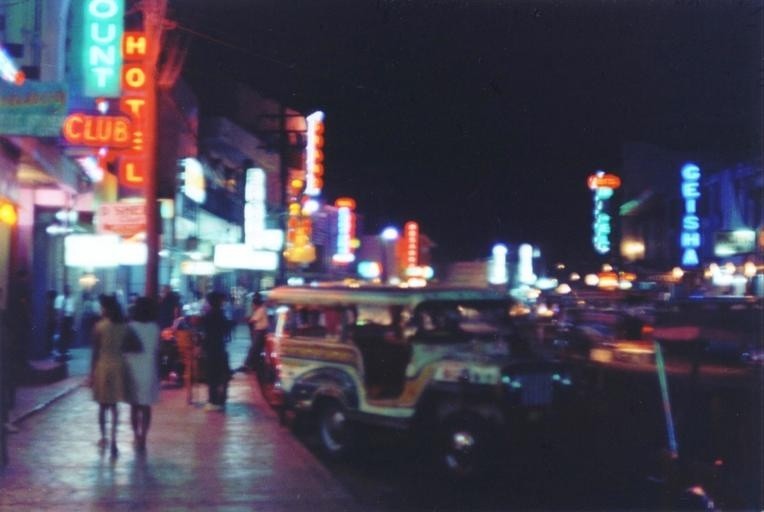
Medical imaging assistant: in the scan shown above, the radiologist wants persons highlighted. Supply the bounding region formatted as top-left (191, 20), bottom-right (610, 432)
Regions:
top-left (87, 295), bottom-right (143, 456)
top-left (50, 285), bottom-right (271, 416)
top-left (123, 295), bottom-right (162, 446)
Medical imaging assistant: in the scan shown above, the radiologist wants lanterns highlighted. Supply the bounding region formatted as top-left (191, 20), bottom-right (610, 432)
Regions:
top-left (407, 223), bottom-right (418, 268)
top-left (282, 174), bottom-right (313, 245)
top-left (310, 119), bottom-right (325, 189)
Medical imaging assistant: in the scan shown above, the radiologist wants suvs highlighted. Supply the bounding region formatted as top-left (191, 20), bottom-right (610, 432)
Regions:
top-left (262, 284), bottom-right (607, 477)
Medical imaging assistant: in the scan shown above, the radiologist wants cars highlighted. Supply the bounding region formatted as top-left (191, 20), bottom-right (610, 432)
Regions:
top-left (509, 291), bottom-right (764, 367)
